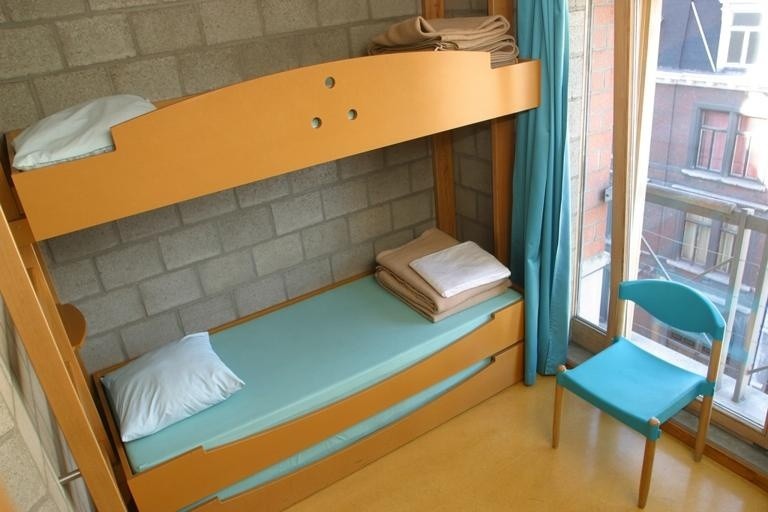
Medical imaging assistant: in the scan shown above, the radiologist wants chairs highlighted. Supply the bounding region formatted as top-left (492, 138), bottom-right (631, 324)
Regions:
top-left (551, 278), bottom-right (725, 510)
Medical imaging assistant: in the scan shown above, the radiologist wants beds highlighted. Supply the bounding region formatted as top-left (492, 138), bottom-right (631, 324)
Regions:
top-left (0, 31), bottom-right (543, 512)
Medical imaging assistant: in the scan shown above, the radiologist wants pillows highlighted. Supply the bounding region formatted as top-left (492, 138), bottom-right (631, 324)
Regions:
top-left (10, 96), bottom-right (158, 171)
top-left (100, 332), bottom-right (246, 443)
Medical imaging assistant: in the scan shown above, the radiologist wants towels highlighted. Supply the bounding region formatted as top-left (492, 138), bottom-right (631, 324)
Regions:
top-left (406, 239), bottom-right (513, 299)
